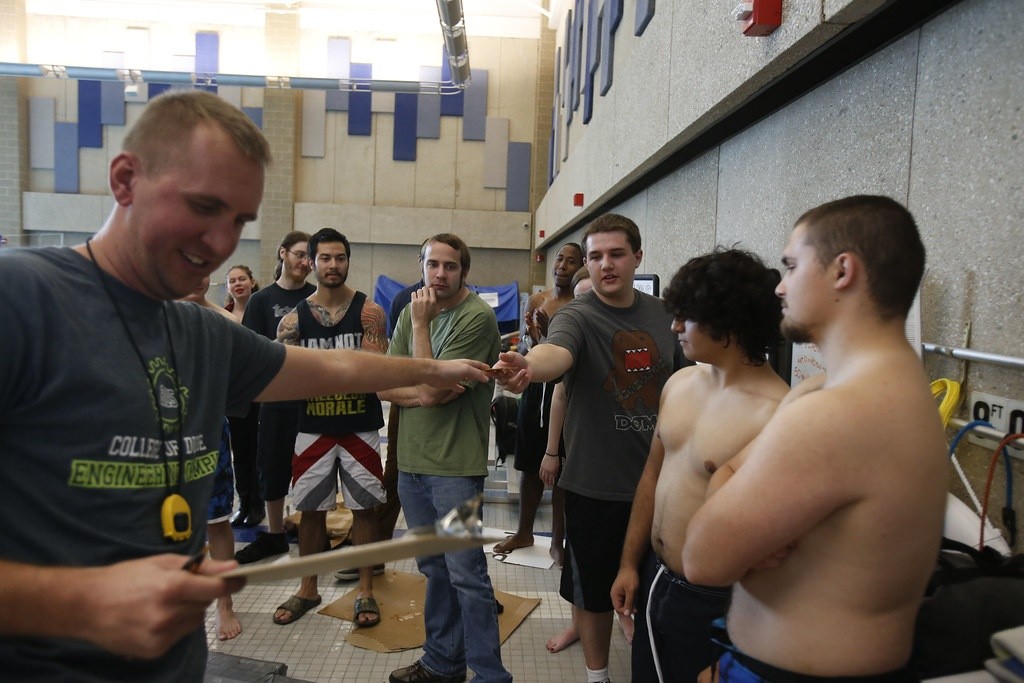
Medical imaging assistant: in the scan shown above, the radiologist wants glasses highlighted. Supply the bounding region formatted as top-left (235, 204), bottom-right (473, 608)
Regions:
top-left (284, 246), bottom-right (311, 262)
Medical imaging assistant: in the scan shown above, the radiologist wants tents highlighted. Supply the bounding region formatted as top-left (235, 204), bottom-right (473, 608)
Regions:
top-left (374, 273), bottom-right (518, 338)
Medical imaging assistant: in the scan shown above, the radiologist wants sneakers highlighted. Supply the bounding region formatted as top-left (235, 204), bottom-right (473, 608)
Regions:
top-left (235, 530), bottom-right (289, 564)
top-left (331, 532), bottom-right (353, 550)
top-left (388, 660), bottom-right (465, 683)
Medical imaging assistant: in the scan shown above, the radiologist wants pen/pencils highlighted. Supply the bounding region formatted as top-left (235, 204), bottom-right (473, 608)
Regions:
top-left (183, 544), bottom-right (211, 573)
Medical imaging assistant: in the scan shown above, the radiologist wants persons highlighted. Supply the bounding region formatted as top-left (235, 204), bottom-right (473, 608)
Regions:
top-left (610, 239), bottom-right (793, 683)
top-left (221, 264), bottom-right (266, 528)
top-left (272, 227), bottom-right (390, 629)
top-left (493, 243), bottom-right (583, 552)
top-left (377, 232), bottom-right (502, 682)
top-left (492, 213), bottom-right (695, 683)
top-left (235, 230), bottom-right (317, 564)
top-left (681, 194), bottom-right (948, 683)
top-left (179, 275), bottom-right (242, 640)
top-left (0, 88), bottom-right (493, 682)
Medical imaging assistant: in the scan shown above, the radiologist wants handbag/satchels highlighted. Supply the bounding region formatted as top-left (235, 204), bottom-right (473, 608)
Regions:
top-left (490, 394), bottom-right (519, 458)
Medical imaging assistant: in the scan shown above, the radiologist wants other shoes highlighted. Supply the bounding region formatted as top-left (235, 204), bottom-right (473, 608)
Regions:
top-left (336, 560), bottom-right (385, 581)
top-left (228, 506), bottom-right (264, 526)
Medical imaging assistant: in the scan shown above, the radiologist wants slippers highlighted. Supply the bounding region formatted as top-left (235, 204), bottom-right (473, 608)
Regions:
top-left (272, 592), bottom-right (323, 625)
top-left (354, 595), bottom-right (380, 626)
